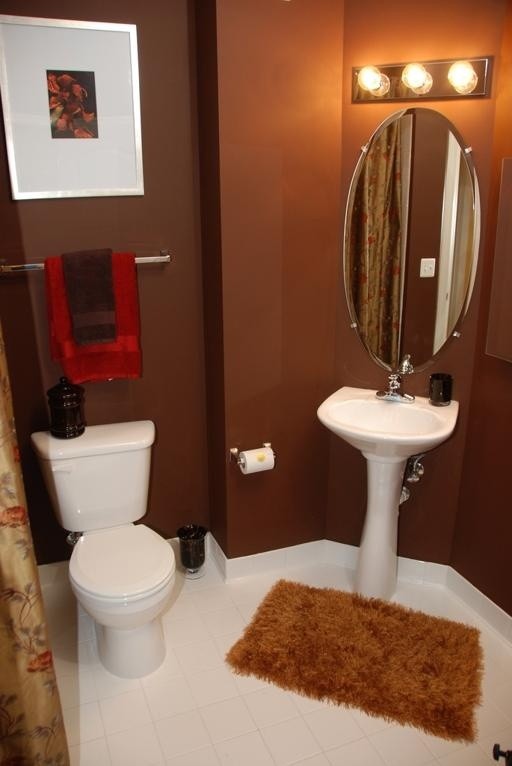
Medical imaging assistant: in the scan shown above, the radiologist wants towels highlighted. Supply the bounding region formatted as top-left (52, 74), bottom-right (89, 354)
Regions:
top-left (47, 250), bottom-right (141, 384)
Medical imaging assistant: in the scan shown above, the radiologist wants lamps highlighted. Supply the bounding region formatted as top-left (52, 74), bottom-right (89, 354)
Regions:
top-left (350, 55), bottom-right (494, 101)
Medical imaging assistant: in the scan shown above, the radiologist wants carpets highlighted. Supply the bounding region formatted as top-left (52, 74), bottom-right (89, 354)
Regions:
top-left (224, 580), bottom-right (483, 747)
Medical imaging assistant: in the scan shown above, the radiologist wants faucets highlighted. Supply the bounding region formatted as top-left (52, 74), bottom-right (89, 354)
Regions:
top-left (375, 373), bottom-right (415, 402)
top-left (399, 354), bottom-right (413, 375)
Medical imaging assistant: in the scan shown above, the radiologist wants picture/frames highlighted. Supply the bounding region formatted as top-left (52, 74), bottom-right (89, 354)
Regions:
top-left (0, 15), bottom-right (146, 200)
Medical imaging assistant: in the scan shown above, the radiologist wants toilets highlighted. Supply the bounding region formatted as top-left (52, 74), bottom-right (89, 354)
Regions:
top-left (28, 420), bottom-right (177, 680)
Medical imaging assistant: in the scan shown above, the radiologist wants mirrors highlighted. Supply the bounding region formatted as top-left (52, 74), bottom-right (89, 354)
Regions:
top-left (342, 108), bottom-right (482, 380)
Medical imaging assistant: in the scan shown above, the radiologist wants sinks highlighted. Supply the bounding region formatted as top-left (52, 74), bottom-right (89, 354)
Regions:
top-left (316, 386), bottom-right (459, 463)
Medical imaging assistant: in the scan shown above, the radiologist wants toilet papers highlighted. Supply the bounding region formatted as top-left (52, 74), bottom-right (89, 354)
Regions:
top-left (237, 447), bottom-right (276, 475)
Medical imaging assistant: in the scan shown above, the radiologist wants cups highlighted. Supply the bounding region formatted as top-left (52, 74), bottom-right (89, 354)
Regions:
top-left (428, 373), bottom-right (453, 406)
top-left (177, 523), bottom-right (208, 580)
